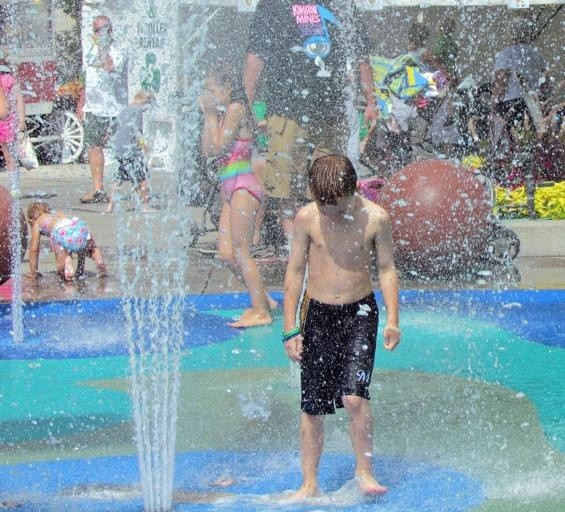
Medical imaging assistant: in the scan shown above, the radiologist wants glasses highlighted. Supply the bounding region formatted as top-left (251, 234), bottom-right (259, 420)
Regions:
top-left (94, 26), bottom-right (109, 33)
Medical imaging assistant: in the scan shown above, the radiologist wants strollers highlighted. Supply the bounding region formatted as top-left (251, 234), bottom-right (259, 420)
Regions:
top-left (180, 90), bottom-right (286, 256)
top-left (357, 58), bottom-right (519, 281)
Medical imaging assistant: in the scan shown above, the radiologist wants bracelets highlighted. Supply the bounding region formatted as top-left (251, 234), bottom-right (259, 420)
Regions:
top-left (282, 328), bottom-right (300, 343)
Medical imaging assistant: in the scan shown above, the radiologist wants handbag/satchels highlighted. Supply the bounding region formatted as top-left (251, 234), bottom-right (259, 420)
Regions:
top-left (493, 179), bottom-right (565, 221)
top-left (16, 130), bottom-right (38, 170)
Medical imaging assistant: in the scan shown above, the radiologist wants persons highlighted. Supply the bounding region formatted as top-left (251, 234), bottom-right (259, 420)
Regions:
top-left (24, 201), bottom-right (110, 284)
top-left (282, 152), bottom-right (401, 500)
top-left (433, 61), bottom-right (456, 90)
top-left (407, 23), bottom-right (437, 65)
top-left (244, 0), bottom-right (381, 283)
top-left (74, 15), bottom-right (127, 205)
top-left (494, 18), bottom-right (548, 141)
top-left (0, 187), bottom-right (27, 286)
top-left (101, 94), bottom-right (162, 213)
top-left (199, 56), bottom-right (278, 330)
top-left (438, 15), bottom-right (459, 68)
top-left (0, 58), bottom-right (26, 171)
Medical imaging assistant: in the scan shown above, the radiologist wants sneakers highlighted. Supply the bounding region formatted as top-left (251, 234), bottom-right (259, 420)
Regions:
top-left (10, 191), bottom-right (23, 200)
top-left (121, 193), bottom-right (140, 199)
top-left (80, 189), bottom-right (107, 202)
